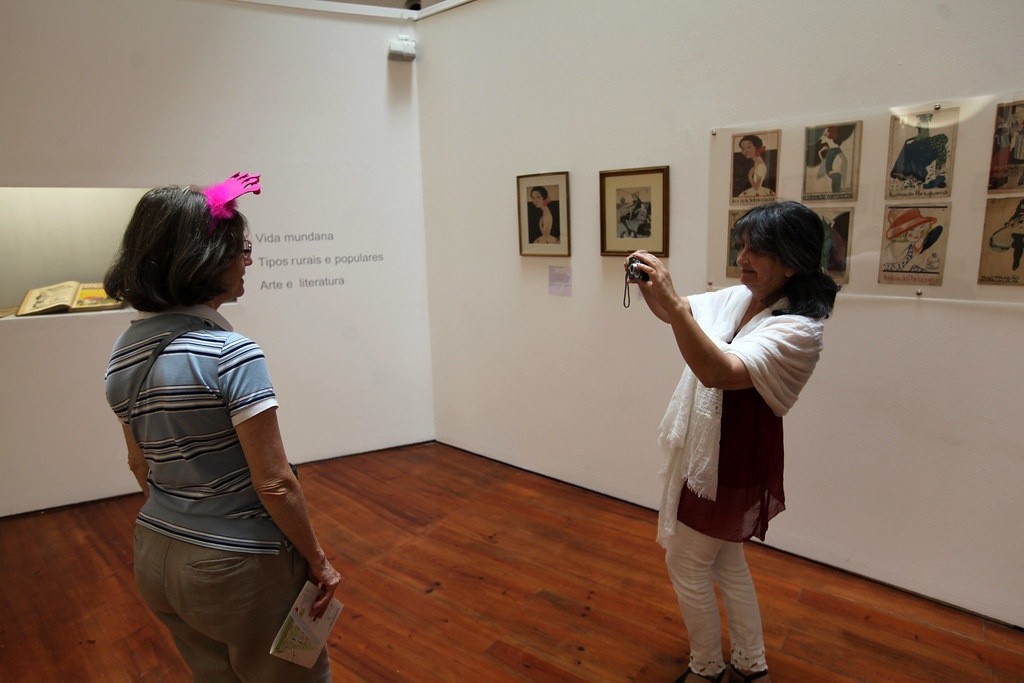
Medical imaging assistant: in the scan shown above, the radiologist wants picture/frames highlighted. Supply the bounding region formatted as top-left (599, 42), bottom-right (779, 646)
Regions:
top-left (599, 165), bottom-right (669, 258)
top-left (516, 171), bottom-right (571, 258)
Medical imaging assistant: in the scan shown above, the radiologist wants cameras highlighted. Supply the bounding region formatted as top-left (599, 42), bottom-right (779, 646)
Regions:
top-left (627, 257), bottom-right (650, 282)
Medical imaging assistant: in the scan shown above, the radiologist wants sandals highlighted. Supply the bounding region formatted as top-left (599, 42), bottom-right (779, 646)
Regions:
top-left (674, 668), bottom-right (732, 683)
top-left (724, 661), bottom-right (771, 682)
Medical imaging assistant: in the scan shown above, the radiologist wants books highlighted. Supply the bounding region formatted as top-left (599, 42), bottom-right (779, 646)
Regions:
top-left (15, 280), bottom-right (130, 318)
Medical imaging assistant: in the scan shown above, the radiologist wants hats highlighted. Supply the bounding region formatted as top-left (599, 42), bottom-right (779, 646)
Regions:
top-left (886, 208), bottom-right (937, 239)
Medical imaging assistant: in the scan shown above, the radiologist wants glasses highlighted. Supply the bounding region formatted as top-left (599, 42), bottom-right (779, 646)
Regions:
top-left (240, 241), bottom-right (252, 260)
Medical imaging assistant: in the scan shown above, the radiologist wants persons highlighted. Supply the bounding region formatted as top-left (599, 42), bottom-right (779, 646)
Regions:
top-left (623, 201), bottom-right (841, 682)
top-left (101, 182), bottom-right (342, 683)
